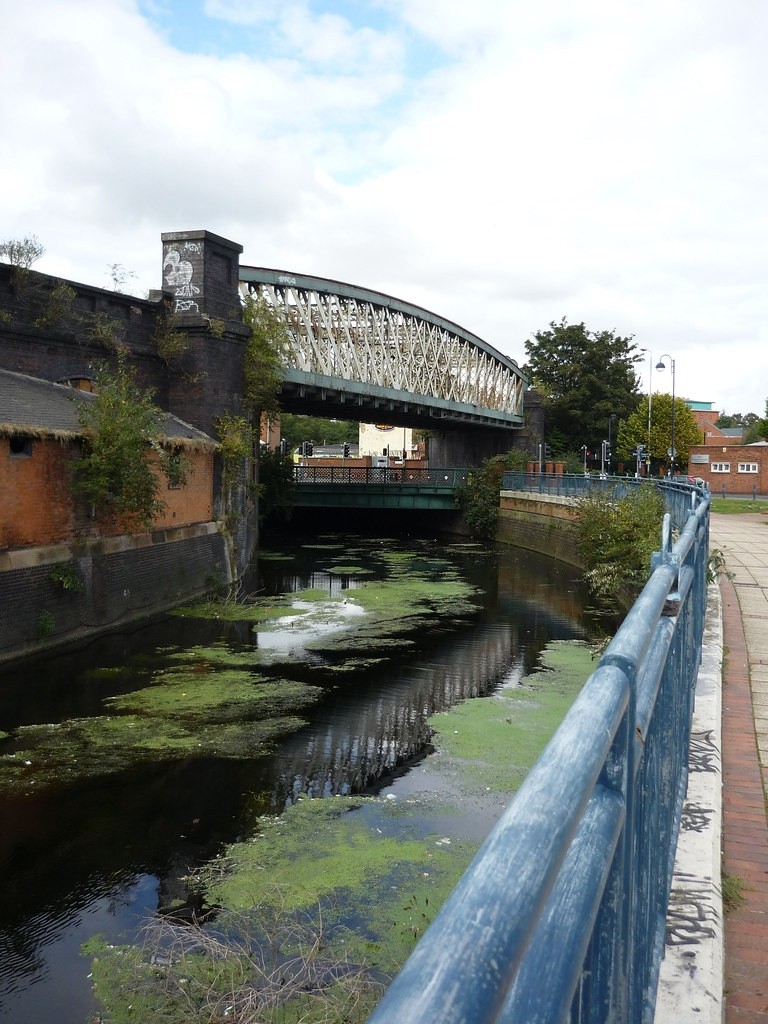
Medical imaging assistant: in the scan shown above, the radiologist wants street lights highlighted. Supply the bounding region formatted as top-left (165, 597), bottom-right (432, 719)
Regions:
top-left (581, 445), bottom-right (587, 474)
top-left (656, 355), bottom-right (675, 480)
top-left (640, 348), bottom-right (652, 476)
top-left (609, 414), bottom-right (617, 474)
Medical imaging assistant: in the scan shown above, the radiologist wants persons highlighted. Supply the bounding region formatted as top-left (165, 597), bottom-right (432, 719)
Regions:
top-left (599, 469), bottom-right (608, 480)
top-left (583, 469), bottom-right (592, 492)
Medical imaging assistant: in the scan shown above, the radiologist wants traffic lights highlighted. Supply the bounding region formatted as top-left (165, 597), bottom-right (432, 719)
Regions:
top-left (284, 441), bottom-right (291, 456)
top-left (309, 445), bottom-right (314, 456)
top-left (605, 442), bottom-right (612, 462)
top-left (306, 443), bottom-right (309, 455)
top-left (543, 443), bottom-right (551, 461)
top-left (345, 444), bottom-right (350, 458)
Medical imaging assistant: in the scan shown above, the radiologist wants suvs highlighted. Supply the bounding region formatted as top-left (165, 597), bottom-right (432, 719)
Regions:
top-left (661, 474), bottom-right (706, 490)
